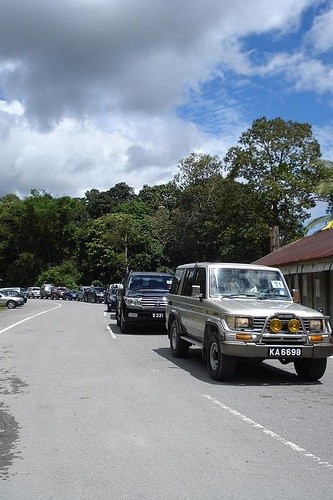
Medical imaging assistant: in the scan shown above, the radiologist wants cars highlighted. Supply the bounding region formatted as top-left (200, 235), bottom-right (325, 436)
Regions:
top-left (0, 293), bottom-right (24, 308)
top-left (0, 288), bottom-right (27, 303)
top-left (64, 284), bottom-right (119, 311)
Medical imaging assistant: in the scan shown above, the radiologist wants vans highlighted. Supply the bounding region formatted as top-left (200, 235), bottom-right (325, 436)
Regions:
top-left (27, 287), bottom-right (40, 298)
top-left (40, 284), bottom-right (54, 299)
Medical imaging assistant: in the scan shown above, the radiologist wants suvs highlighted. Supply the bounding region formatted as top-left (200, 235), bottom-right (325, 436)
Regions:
top-left (51, 287), bottom-right (66, 299)
top-left (115, 269), bottom-right (173, 334)
top-left (164, 260), bottom-right (333, 381)
